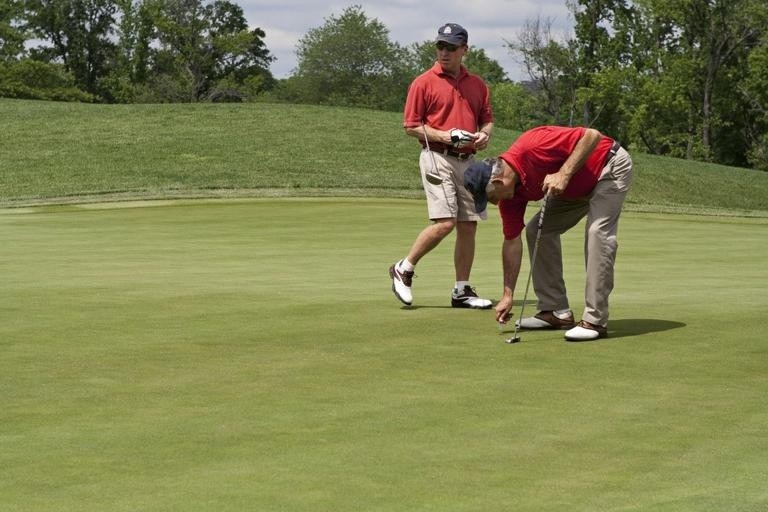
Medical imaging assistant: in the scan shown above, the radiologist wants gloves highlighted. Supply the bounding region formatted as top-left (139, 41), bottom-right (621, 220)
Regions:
top-left (450, 128), bottom-right (477, 149)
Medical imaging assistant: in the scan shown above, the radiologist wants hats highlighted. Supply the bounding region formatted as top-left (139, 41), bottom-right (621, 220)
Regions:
top-left (436, 23), bottom-right (468, 45)
top-left (463, 162), bottom-right (492, 213)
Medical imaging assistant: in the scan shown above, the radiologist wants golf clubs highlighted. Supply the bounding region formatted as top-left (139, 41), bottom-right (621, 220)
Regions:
top-left (421, 120), bottom-right (443, 186)
top-left (504, 190), bottom-right (550, 343)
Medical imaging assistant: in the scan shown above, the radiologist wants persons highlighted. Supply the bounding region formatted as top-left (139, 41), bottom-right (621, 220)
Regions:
top-left (462, 125), bottom-right (633, 341)
top-left (389, 22), bottom-right (495, 309)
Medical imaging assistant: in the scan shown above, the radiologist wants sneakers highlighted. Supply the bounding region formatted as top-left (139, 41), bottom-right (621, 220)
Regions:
top-left (515, 309), bottom-right (575, 330)
top-left (389, 259), bottom-right (414, 305)
top-left (451, 285), bottom-right (492, 309)
top-left (564, 320), bottom-right (608, 341)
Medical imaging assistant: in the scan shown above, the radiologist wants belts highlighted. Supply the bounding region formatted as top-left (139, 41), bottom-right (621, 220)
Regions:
top-left (423, 145), bottom-right (469, 159)
top-left (603, 143), bottom-right (621, 167)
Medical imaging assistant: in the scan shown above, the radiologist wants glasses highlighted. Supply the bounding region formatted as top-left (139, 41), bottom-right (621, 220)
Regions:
top-left (436, 43), bottom-right (463, 52)
